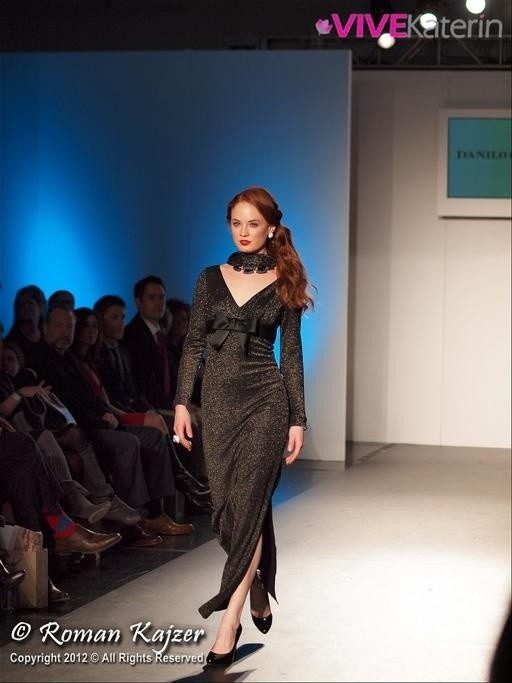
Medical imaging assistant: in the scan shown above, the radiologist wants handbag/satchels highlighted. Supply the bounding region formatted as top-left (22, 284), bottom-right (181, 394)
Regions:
top-left (0, 524), bottom-right (48, 611)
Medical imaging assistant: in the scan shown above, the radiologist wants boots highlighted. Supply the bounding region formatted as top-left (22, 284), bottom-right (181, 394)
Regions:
top-left (164, 434), bottom-right (211, 504)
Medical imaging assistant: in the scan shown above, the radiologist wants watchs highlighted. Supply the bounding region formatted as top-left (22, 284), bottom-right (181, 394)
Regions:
top-left (15, 390), bottom-right (26, 399)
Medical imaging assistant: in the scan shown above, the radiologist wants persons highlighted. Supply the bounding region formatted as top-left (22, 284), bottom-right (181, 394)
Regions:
top-left (26, 306), bottom-right (193, 548)
top-left (70, 308), bottom-right (211, 508)
top-left (94, 295), bottom-right (211, 515)
top-left (119, 276), bottom-right (207, 479)
top-left (1, 340), bottom-right (146, 524)
top-left (161, 308), bottom-right (172, 334)
top-left (173, 187), bottom-right (318, 664)
top-left (0, 431), bottom-right (121, 603)
top-left (166, 299), bottom-right (205, 407)
top-left (10, 287), bottom-right (46, 354)
top-left (49, 290), bottom-right (74, 311)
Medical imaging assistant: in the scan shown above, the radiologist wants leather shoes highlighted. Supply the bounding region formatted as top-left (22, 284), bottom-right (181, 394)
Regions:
top-left (251, 570), bottom-right (272, 634)
top-left (145, 512), bottom-right (193, 536)
top-left (186, 496), bottom-right (213, 513)
top-left (54, 524), bottom-right (121, 556)
top-left (121, 525), bottom-right (164, 546)
top-left (48, 573), bottom-right (70, 603)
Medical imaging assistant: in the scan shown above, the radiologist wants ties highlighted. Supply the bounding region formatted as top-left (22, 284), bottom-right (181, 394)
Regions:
top-left (156, 330), bottom-right (170, 392)
top-left (113, 346), bottom-right (124, 380)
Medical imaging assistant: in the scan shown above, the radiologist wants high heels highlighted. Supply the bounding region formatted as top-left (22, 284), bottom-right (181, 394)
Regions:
top-left (206, 624), bottom-right (242, 666)
top-left (69, 498), bottom-right (111, 525)
top-left (106, 496), bottom-right (147, 526)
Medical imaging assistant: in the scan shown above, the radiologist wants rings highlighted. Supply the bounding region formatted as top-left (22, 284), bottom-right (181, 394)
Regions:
top-left (173, 434), bottom-right (180, 443)
